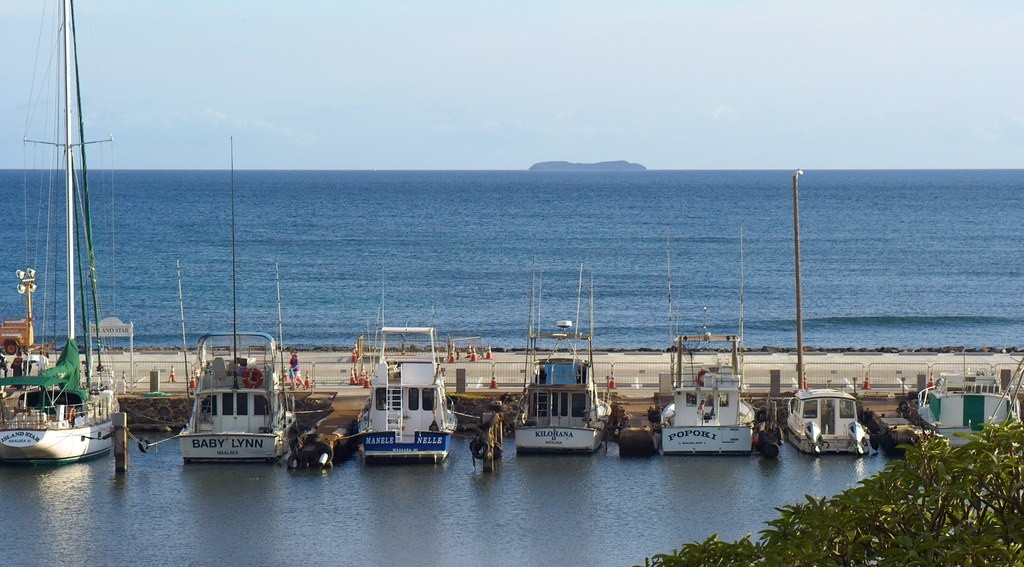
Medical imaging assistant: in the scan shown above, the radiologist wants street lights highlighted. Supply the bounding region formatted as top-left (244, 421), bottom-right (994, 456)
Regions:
top-left (793, 169), bottom-right (804, 390)
top-left (17, 267), bottom-right (36, 345)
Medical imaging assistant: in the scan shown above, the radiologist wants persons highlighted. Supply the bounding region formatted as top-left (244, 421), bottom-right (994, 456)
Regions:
top-left (37, 351), bottom-right (49, 376)
top-left (0, 350), bottom-right (8, 378)
top-left (11, 351), bottom-right (32, 377)
top-left (289, 351), bottom-right (307, 390)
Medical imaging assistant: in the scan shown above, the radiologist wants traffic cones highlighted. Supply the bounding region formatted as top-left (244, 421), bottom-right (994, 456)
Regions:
top-left (490, 371), bottom-right (499, 390)
top-left (169, 364), bottom-right (175, 385)
top-left (348, 365), bottom-right (373, 388)
top-left (928, 372), bottom-right (934, 388)
top-left (804, 371), bottom-right (807, 389)
top-left (303, 370), bottom-right (311, 389)
top-left (190, 370), bottom-right (196, 389)
top-left (472, 347), bottom-right (477, 361)
top-left (353, 342), bottom-right (359, 362)
top-left (450, 351), bottom-right (456, 364)
top-left (487, 345), bottom-right (493, 359)
top-left (609, 370), bottom-right (617, 390)
top-left (864, 372), bottom-right (870, 389)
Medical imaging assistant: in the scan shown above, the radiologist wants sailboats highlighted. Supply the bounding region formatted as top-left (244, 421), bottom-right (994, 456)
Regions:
top-left (0, 0), bottom-right (120, 468)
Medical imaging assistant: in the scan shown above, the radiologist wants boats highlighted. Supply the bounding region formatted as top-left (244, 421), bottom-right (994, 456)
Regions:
top-left (917, 385), bottom-right (1021, 446)
top-left (659, 308), bottom-right (756, 453)
top-left (786, 389), bottom-right (870, 456)
top-left (360, 324), bottom-right (458, 461)
top-left (180, 137), bottom-right (293, 465)
top-left (514, 321), bottom-right (612, 452)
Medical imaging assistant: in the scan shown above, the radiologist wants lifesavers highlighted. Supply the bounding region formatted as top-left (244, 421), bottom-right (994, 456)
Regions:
top-left (139, 437), bottom-right (151, 452)
top-left (68, 407), bottom-right (77, 426)
top-left (696, 370), bottom-right (711, 385)
top-left (242, 367), bottom-right (263, 388)
top-left (314, 442), bottom-right (333, 465)
top-left (899, 400), bottom-right (909, 410)
top-left (470, 437), bottom-right (489, 457)
top-left (763, 443), bottom-right (780, 457)
